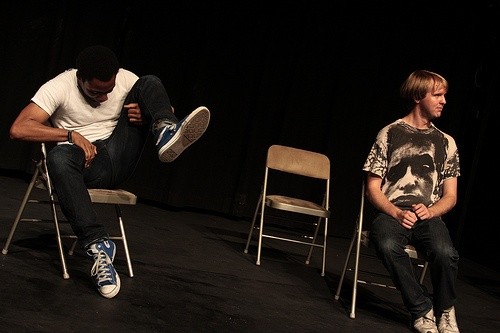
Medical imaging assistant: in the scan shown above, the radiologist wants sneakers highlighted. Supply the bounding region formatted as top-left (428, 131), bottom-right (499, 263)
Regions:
top-left (154, 106), bottom-right (210, 163)
top-left (438, 305), bottom-right (460, 333)
top-left (414, 305), bottom-right (439, 333)
top-left (86, 240), bottom-right (121, 299)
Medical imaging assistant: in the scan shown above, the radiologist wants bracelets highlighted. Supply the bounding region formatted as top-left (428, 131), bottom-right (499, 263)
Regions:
top-left (68, 130), bottom-right (74, 143)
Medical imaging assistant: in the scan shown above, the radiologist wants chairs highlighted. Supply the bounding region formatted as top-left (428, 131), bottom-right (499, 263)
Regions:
top-left (335, 172), bottom-right (430, 319)
top-left (244, 144), bottom-right (333, 277)
top-left (2, 139), bottom-right (137, 281)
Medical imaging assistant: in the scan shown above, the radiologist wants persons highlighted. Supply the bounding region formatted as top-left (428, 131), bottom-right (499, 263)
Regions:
top-left (10, 46), bottom-right (210, 298)
top-left (362, 69), bottom-right (459, 333)
